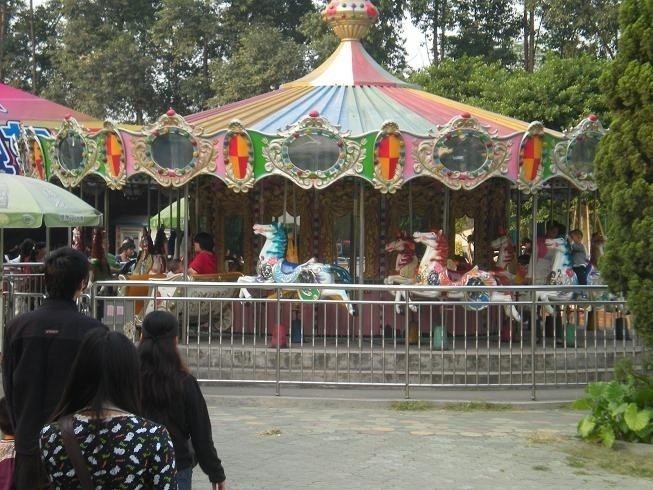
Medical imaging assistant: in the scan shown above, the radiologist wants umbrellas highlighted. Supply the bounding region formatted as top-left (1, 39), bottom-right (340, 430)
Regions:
top-left (0, 170), bottom-right (104, 340)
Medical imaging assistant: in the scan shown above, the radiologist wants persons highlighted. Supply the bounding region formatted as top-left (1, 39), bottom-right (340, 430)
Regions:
top-left (132, 310), bottom-right (226, 490)
top-left (518, 221), bottom-right (588, 285)
top-left (3, 238), bottom-right (47, 310)
top-left (39, 327), bottom-right (179, 489)
top-left (116, 232), bottom-right (217, 274)
top-left (0, 246), bottom-right (110, 490)
top-left (0, 397), bottom-right (17, 490)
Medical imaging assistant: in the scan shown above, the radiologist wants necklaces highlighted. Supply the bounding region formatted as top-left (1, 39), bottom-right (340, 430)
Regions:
top-left (78, 404), bottom-right (127, 414)
